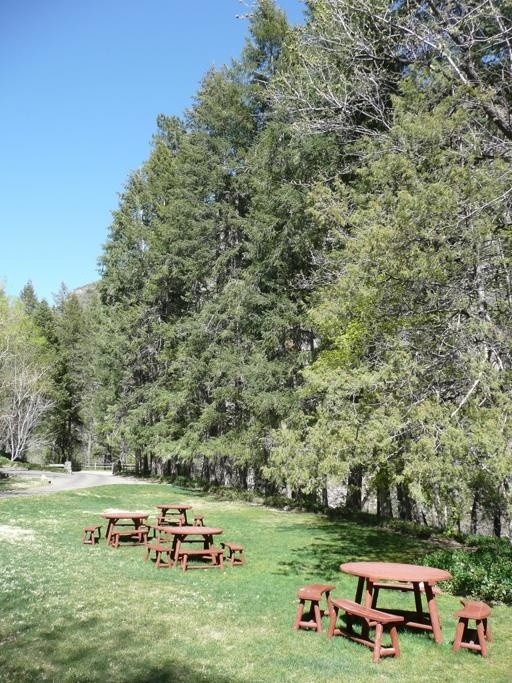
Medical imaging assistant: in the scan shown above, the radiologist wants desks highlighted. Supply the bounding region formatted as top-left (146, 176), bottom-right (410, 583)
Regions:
top-left (102, 503), bottom-right (192, 546)
top-left (339, 561), bottom-right (453, 644)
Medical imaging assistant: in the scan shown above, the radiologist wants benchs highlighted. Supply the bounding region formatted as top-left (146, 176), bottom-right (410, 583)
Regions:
top-left (81, 514), bottom-right (246, 576)
top-left (294, 581), bottom-right (493, 664)
